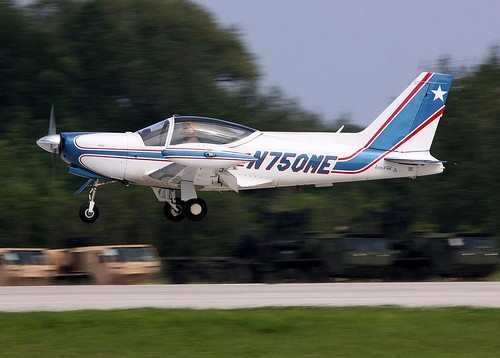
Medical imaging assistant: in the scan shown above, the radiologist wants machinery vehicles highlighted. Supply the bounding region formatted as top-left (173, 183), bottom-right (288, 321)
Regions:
top-left (0, 232), bottom-right (499, 286)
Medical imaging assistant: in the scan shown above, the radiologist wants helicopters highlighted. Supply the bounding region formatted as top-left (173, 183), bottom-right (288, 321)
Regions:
top-left (35, 72), bottom-right (453, 222)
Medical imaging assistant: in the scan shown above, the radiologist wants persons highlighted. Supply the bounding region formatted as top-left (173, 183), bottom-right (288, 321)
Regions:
top-left (179, 121), bottom-right (201, 143)
